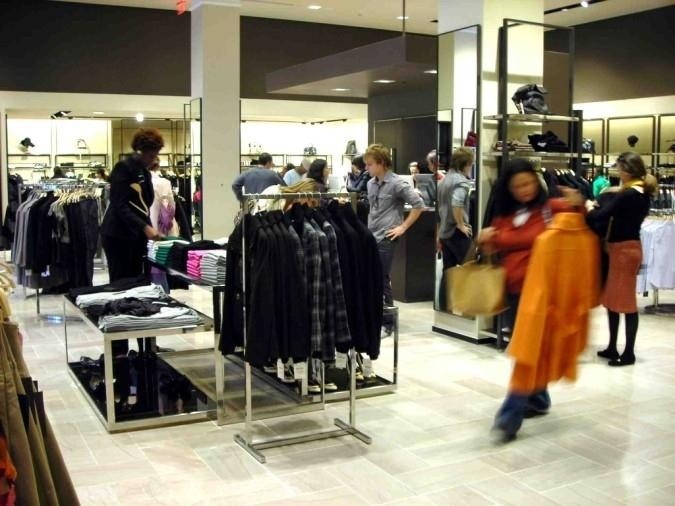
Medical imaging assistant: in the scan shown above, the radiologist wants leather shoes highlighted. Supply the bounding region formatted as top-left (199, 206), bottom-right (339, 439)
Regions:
top-left (281, 368), bottom-right (295, 383)
top-left (597, 350), bottom-right (619, 359)
top-left (319, 378), bottom-right (338, 390)
top-left (357, 352), bottom-right (376, 377)
top-left (489, 425), bottom-right (516, 444)
top-left (308, 379), bottom-right (322, 392)
top-left (608, 355), bottom-right (635, 365)
top-left (347, 355), bottom-right (364, 379)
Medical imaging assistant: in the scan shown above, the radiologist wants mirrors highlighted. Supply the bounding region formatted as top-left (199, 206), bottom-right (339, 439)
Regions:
top-left (434, 23), bottom-right (480, 320)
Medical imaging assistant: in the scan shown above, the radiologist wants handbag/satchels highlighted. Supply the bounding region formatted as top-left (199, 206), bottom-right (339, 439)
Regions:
top-left (512, 82), bottom-right (550, 114)
top-left (445, 242), bottom-right (508, 314)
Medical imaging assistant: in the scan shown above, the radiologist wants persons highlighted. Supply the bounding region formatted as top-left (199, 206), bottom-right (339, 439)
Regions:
top-left (148, 156), bottom-right (176, 237)
top-left (362, 142), bottom-right (426, 342)
top-left (477, 155), bottom-right (589, 448)
top-left (434, 147), bottom-right (474, 316)
top-left (51, 165), bottom-right (68, 181)
top-left (99, 127), bottom-right (164, 358)
top-left (232, 152), bottom-right (329, 211)
top-left (160, 161), bottom-right (201, 242)
top-left (346, 149), bottom-right (447, 204)
top-left (95, 168), bottom-right (105, 180)
top-left (583, 148), bottom-right (654, 370)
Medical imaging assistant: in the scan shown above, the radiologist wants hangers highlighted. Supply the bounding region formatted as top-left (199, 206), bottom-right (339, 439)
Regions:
top-left (16, 183), bottom-right (100, 212)
top-left (230, 191), bottom-right (370, 240)
